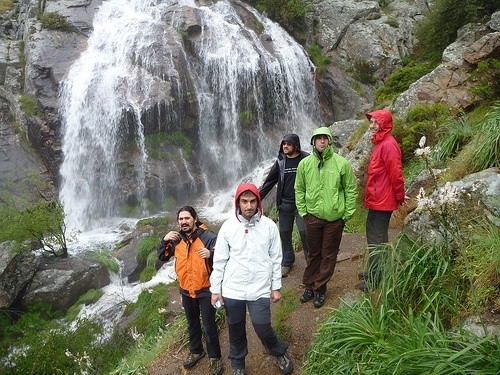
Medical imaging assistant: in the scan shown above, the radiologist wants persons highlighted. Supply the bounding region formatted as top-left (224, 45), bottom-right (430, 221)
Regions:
top-left (356, 110), bottom-right (407, 293)
top-left (258, 133), bottom-right (311, 277)
top-left (209, 183), bottom-right (294, 375)
top-left (158, 206), bottom-right (224, 375)
top-left (294, 127), bottom-right (355, 308)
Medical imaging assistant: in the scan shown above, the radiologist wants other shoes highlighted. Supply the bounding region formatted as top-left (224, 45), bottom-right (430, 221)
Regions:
top-left (358, 272), bottom-right (374, 283)
top-left (281, 266), bottom-right (292, 277)
top-left (356, 283), bottom-right (378, 293)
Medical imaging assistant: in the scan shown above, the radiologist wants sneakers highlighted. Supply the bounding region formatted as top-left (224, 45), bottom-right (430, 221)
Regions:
top-left (276, 351), bottom-right (293, 375)
top-left (208, 358), bottom-right (224, 375)
top-left (300, 288), bottom-right (314, 303)
top-left (183, 353), bottom-right (202, 368)
top-left (312, 292), bottom-right (327, 307)
top-left (232, 367), bottom-right (245, 375)
